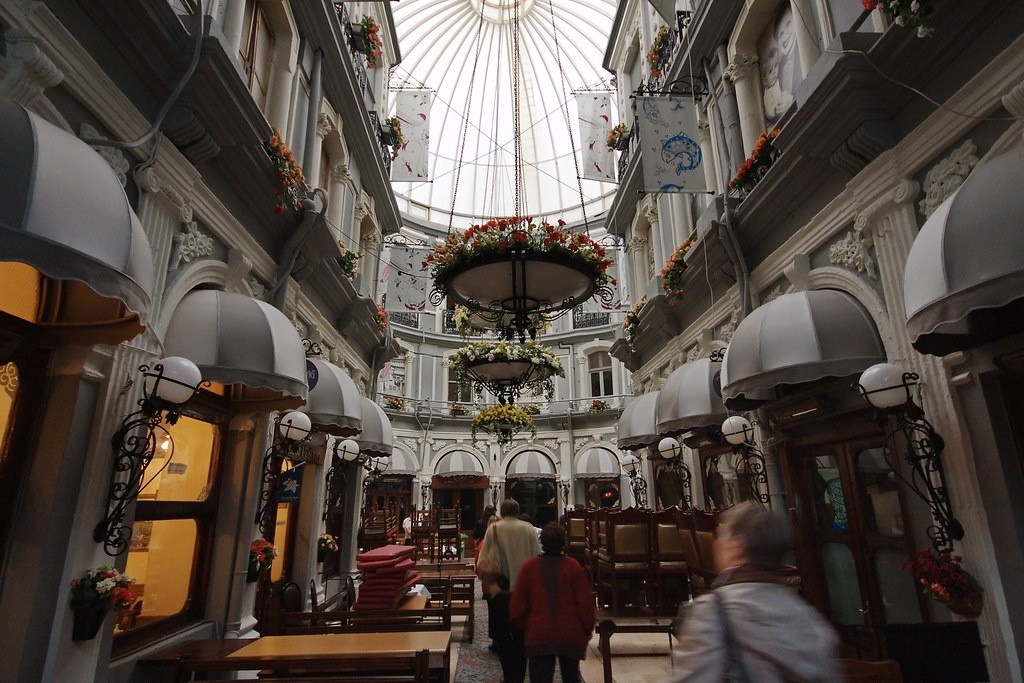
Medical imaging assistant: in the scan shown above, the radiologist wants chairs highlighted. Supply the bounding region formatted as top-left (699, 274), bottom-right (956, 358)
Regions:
top-left (565, 495), bottom-right (736, 619)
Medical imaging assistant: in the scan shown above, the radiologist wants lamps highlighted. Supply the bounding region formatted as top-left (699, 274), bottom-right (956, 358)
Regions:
top-left (360, 456), bottom-right (392, 518)
top-left (322, 439), bottom-right (361, 522)
top-left (716, 415), bottom-right (771, 507)
top-left (656, 437), bottom-right (694, 511)
top-left (93, 356), bottom-right (211, 556)
top-left (849, 362), bottom-right (965, 554)
top-left (254, 411), bottom-right (319, 539)
top-left (622, 455), bottom-right (647, 507)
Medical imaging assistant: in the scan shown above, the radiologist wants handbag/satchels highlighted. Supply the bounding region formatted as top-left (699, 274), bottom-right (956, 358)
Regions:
top-left (474, 522), bottom-right (503, 582)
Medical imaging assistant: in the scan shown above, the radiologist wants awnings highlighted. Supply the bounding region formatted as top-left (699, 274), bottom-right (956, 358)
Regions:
top-left (617, 150), bottom-right (1024, 451)
top-left (369, 448), bottom-right (620, 479)
top-left (0, 97), bottom-right (394, 458)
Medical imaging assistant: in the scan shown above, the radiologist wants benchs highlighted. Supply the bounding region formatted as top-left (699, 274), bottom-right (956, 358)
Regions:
top-left (277, 576), bottom-right (474, 643)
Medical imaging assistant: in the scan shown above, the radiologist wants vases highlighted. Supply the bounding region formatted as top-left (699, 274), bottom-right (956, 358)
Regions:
top-left (72, 601), bottom-right (110, 641)
top-left (615, 131), bottom-right (629, 150)
top-left (318, 550), bottom-right (327, 562)
top-left (247, 560), bottom-right (259, 583)
top-left (448, 263), bottom-right (596, 314)
top-left (481, 425), bottom-right (513, 430)
top-left (351, 22), bottom-right (367, 54)
top-left (463, 360), bottom-right (555, 385)
top-left (944, 593), bottom-right (982, 619)
top-left (383, 124), bottom-right (395, 146)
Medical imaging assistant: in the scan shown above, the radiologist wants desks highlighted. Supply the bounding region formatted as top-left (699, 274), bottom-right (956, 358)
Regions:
top-left (135, 630), bottom-right (454, 683)
top-left (360, 498), bottom-right (462, 563)
top-left (418, 569), bottom-right (477, 644)
top-left (348, 594), bottom-right (428, 624)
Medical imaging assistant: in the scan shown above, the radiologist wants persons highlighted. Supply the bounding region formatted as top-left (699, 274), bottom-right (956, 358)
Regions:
top-left (509, 522), bottom-right (596, 683)
top-left (403, 513), bottom-right (415, 546)
top-left (758, 0), bottom-right (803, 125)
top-left (478, 499), bottom-right (541, 683)
top-left (473, 504), bottom-right (532, 651)
top-left (657, 501), bottom-right (842, 683)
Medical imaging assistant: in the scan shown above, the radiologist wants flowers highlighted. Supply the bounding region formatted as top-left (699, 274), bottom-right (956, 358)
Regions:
top-left (386, 396), bottom-right (406, 414)
top-left (249, 538), bottom-right (279, 572)
top-left (861, 0), bottom-right (936, 38)
top-left (605, 123), bottom-right (627, 152)
top-left (360, 14), bottom-right (384, 69)
top-left (385, 117), bottom-right (406, 161)
top-left (318, 533), bottom-right (338, 553)
top-left (899, 547), bottom-right (982, 601)
top-left (621, 297), bottom-right (646, 353)
top-left (337, 237), bottom-right (366, 284)
top-left (374, 303), bottom-right (390, 332)
top-left (728, 128), bottom-right (781, 189)
top-left (421, 217), bottom-right (617, 444)
top-left (69, 566), bottom-right (137, 613)
top-left (645, 25), bottom-right (668, 78)
top-left (263, 127), bottom-right (306, 215)
top-left (659, 238), bottom-right (697, 306)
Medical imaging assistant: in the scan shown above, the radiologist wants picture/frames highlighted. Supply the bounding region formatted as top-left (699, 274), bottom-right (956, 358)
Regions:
top-left (128, 493), bottom-right (155, 553)
